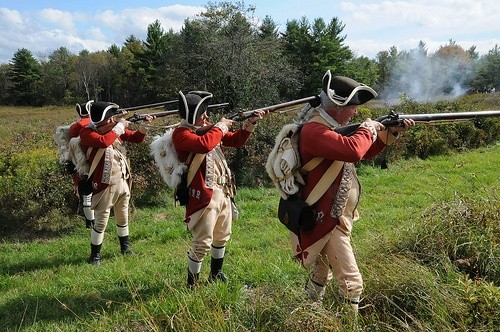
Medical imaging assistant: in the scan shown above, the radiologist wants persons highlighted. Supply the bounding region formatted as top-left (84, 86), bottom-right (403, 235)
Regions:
top-left (69, 100), bottom-right (95, 228)
top-left (78, 101), bottom-right (156, 265)
top-left (291, 77), bottom-right (415, 324)
top-left (172, 90), bottom-right (270, 287)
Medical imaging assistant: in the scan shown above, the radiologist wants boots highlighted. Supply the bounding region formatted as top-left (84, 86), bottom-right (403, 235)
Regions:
top-left (206, 257), bottom-right (229, 285)
top-left (86, 219), bottom-right (95, 229)
top-left (188, 268), bottom-right (201, 291)
top-left (89, 243), bottom-right (103, 267)
top-left (118, 234), bottom-right (135, 256)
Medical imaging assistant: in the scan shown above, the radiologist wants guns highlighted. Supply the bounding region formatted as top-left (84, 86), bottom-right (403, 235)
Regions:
top-left (114, 99), bottom-right (179, 114)
top-left (95, 102), bottom-right (230, 135)
top-left (333, 108), bottom-right (500, 139)
top-left (196, 93), bottom-right (320, 136)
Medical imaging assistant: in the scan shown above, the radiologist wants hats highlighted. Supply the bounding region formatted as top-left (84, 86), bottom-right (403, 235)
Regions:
top-left (321, 70), bottom-right (378, 107)
top-left (75, 102), bottom-right (89, 117)
top-left (178, 89), bottom-right (213, 126)
top-left (86, 99), bottom-right (119, 124)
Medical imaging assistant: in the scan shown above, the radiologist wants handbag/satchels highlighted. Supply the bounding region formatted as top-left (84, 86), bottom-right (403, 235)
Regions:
top-left (277, 194), bottom-right (317, 235)
top-left (65, 161), bottom-right (76, 174)
top-left (77, 181), bottom-right (93, 196)
